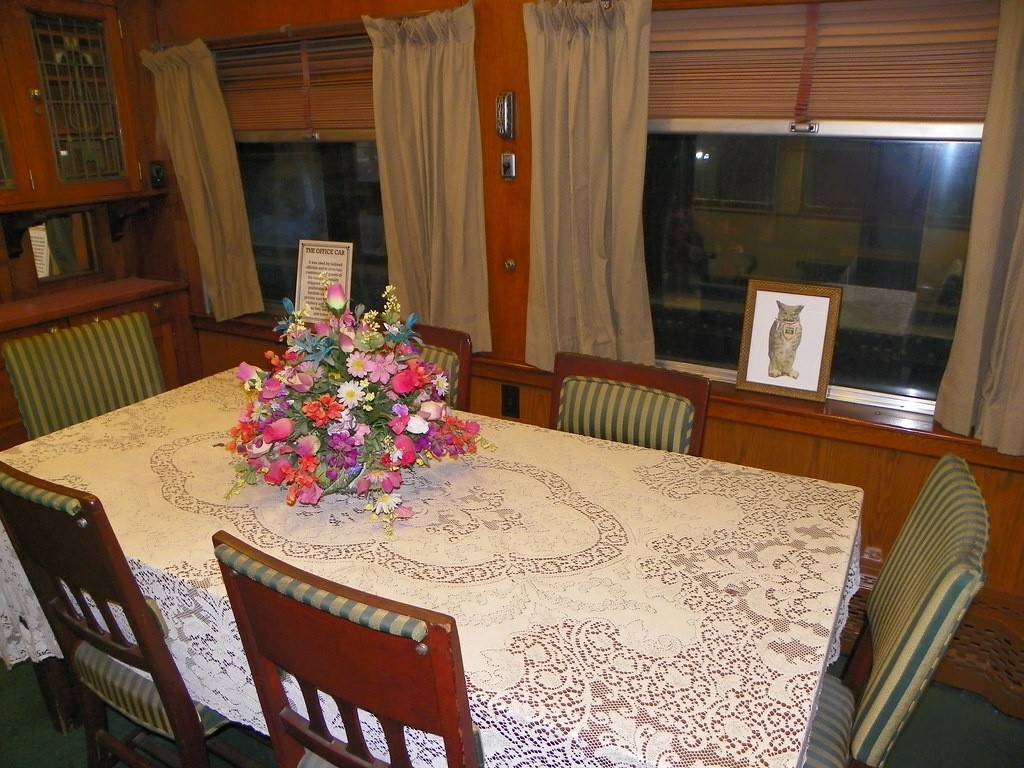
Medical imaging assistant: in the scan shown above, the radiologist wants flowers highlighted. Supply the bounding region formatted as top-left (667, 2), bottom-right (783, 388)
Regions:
top-left (221, 270), bottom-right (483, 543)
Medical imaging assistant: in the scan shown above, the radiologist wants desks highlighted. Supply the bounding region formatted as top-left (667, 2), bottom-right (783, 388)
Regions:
top-left (0, 360), bottom-right (866, 768)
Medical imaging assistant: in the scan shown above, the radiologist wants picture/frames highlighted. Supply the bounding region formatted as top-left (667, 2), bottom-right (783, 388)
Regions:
top-left (736, 279), bottom-right (843, 403)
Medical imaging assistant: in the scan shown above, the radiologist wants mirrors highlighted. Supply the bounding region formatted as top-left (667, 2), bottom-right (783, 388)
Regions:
top-left (27, 211), bottom-right (103, 284)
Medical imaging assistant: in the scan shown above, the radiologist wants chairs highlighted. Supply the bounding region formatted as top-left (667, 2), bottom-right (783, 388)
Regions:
top-left (801, 450), bottom-right (991, 768)
top-left (374, 318), bottom-right (472, 412)
top-left (3, 312), bottom-right (169, 440)
top-left (0, 461), bottom-right (273, 768)
top-left (210, 530), bottom-right (480, 768)
top-left (547, 352), bottom-right (711, 458)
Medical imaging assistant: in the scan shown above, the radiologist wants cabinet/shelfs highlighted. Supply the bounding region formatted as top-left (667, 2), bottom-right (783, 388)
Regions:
top-left (0, 0), bottom-right (171, 214)
top-left (0, 278), bottom-right (202, 452)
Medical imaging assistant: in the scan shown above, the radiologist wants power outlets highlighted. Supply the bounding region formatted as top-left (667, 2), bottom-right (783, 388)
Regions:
top-left (501, 384), bottom-right (520, 419)
